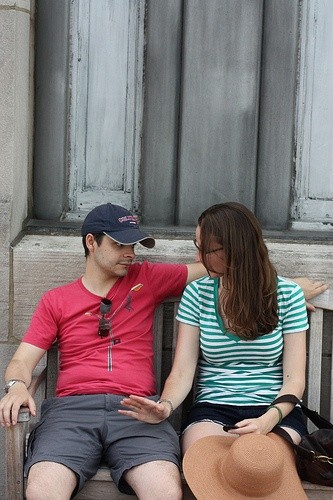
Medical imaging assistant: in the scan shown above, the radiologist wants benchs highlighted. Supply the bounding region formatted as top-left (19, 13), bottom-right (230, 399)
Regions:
top-left (2, 289), bottom-right (333, 500)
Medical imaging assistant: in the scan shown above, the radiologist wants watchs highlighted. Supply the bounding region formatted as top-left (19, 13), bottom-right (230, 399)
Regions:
top-left (3, 379), bottom-right (26, 393)
top-left (156, 399), bottom-right (173, 415)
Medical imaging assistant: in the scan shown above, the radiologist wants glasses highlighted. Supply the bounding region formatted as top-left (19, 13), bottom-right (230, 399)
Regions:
top-left (97, 298), bottom-right (113, 337)
top-left (194, 240), bottom-right (224, 255)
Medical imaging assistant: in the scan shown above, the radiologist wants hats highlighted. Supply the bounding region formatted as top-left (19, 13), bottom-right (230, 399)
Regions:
top-left (181, 432), bottom-right (302, 499)
top-left (81, 201), bottom-right (156, 249)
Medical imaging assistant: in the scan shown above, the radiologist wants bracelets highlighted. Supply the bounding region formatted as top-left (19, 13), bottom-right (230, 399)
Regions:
top-left (266, 405), bottom-right (283, 424)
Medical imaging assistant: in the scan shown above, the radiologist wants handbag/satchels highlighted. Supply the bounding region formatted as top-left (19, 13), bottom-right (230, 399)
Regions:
top-left (267, 394), bottom-right (333, 488)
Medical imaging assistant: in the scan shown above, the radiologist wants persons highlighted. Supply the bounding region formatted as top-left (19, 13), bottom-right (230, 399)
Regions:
top-left (0, 203), bottom-right (329, 500)
top-left (118, 201), bottom-right (309, 500)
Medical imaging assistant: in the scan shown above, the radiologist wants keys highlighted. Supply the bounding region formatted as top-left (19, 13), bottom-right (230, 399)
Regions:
top-left (213, 420), bottom-right (240, 432)
